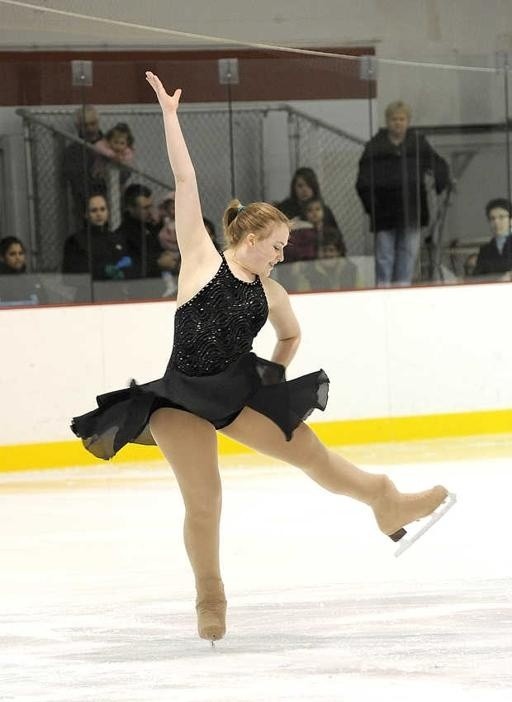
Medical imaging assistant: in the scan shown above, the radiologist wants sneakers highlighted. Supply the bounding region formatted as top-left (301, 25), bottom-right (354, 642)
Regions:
top-left (163, 282), bottom-right (176, 298)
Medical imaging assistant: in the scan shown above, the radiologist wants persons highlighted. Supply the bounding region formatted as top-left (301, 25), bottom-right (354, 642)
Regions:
top-left (0, 236), bottom-right (29, 274)
top-left (62, 105), bottom-right (222, 298)
top-left (283, 196), bottom-right (345, 263)
top-left (354, 101), bottom-right (452, 286)
top-left (273, 163), bottom-right (346, 263)
top-left (69, 68), bottom-right (448, 641)
top-left (475, 198), bottom-right (512, 276)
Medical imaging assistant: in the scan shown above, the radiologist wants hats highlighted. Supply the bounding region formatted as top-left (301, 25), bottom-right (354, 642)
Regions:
top-left (160, 190), bottom-right (175, 204)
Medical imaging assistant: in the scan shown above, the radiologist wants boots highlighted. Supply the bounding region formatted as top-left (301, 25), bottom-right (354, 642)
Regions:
top-left (195, 578), bottom-right (228, 643)
top-left (363, 476), bottom-right (448, 542)
